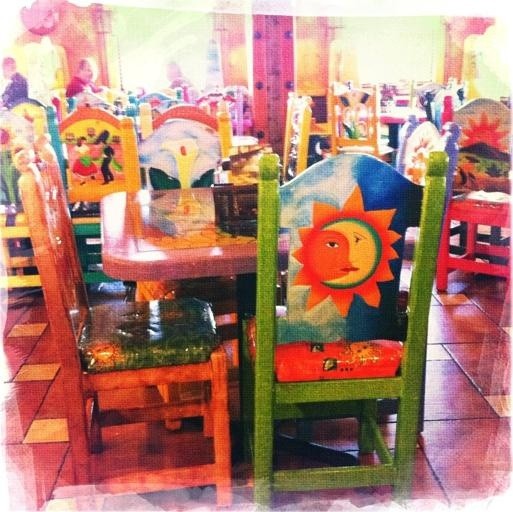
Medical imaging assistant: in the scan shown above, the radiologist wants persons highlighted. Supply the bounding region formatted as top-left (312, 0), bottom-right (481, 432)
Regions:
top-left (165, 60), bottom-right (196, 90)
top-left (0, 57), bottom-right (29, 110)
top-left (66, 57), bottom-right (106, 97)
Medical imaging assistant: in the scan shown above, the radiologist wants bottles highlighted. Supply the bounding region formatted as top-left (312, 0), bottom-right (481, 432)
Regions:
top-left (218, 160), bottom-right (233, 185)
